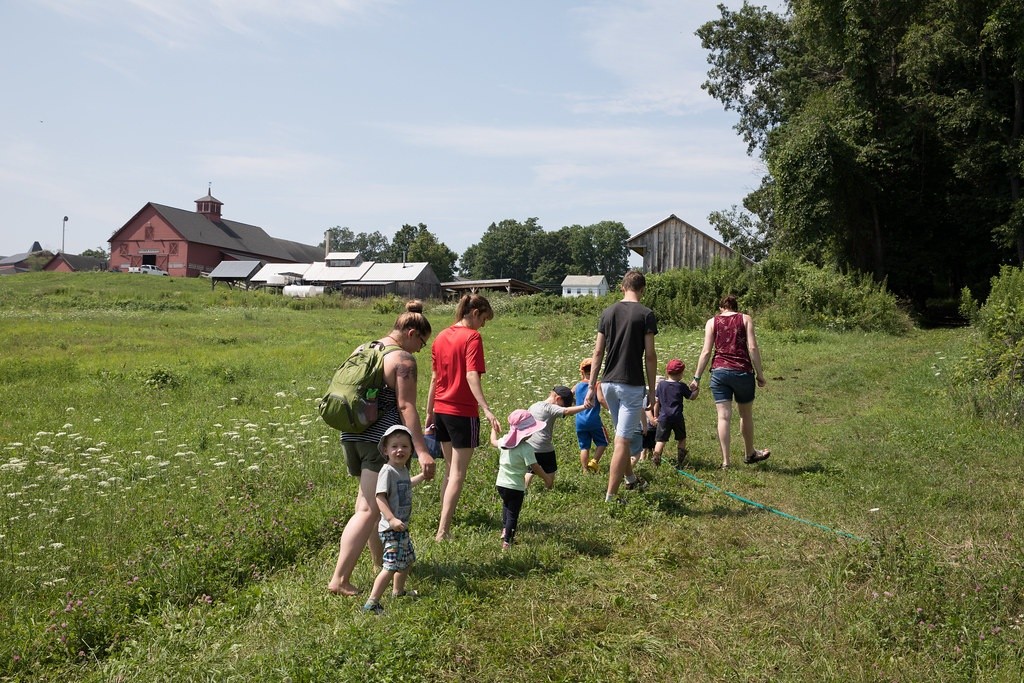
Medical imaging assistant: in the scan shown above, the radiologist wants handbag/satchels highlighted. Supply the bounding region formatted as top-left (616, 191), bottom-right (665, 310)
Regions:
top-left (412, 425), bottom-right (444, 458)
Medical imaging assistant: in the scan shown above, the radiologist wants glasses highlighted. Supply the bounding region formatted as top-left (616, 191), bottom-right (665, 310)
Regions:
top-left (408, 328), bottom-right (427, 349)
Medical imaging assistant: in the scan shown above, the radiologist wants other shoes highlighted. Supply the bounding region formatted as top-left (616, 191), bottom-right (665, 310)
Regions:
top-left (500, 527), bottom-right (506, 539)
top-left (625, 476), bottom-right (647, 492)
top-left (392, 591), bottom-right (415, 598)
top-left (675, 465), bottom-right (684, 470)
top-left (502, 540), bottom-right (509, 549)
top-left (652, 453), bottom-right (662, 466)
top-left (587, 458), bottom-right (601, 474)
top-left (605, 498), bottom-right (628, 505)
top-left (363, 603), bottom-right (385, 611)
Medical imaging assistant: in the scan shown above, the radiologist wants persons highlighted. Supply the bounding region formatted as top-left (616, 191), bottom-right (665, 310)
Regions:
top-left (639, 358), bottom-right (700, 470)
top-left (524, 386), bottom-right (594, 491)
top-left (491, 409), bottom-right (554, 549)
top-left (364, 424), bottom-right (426, 615)
top-left (327, 299), bottom-right (435, 595)
top-left (584, 270), bottom-right (658, 506)
top-left (690, 296), bottom-right (771, 471)
top-left (425, 289), bottom-right (501, 543)
top-left (571, 357), bottom-right (610, 479)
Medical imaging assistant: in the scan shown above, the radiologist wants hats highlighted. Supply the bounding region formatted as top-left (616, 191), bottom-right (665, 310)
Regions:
top-left (553, 386), bottom-right (574, 418)
top-left (666, 359), bottom-right (685, 374)
top-left (377, 424), bottom-right (415, 462)
top-left (500, 409), bottom-right (547, 450)
top-left (580, 358), bottom-right (593, 370)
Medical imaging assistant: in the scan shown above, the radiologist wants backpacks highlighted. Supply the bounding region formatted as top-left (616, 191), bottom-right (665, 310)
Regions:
top-left (319, 341), bottom-right (403, 432)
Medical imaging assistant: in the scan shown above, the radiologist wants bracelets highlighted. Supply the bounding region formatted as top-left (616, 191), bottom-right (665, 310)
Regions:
top-left (692, 376), bottom-right (701, 381)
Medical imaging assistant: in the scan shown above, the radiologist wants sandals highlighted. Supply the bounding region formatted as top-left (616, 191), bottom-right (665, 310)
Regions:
top-left (717, 464), bottom-right (729, 471)
top-left (743, 448), bottom-right (771, 464)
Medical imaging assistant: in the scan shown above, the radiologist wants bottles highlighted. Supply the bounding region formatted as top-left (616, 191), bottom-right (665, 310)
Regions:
top-left (366, 388), bottom-right (378, 421)
